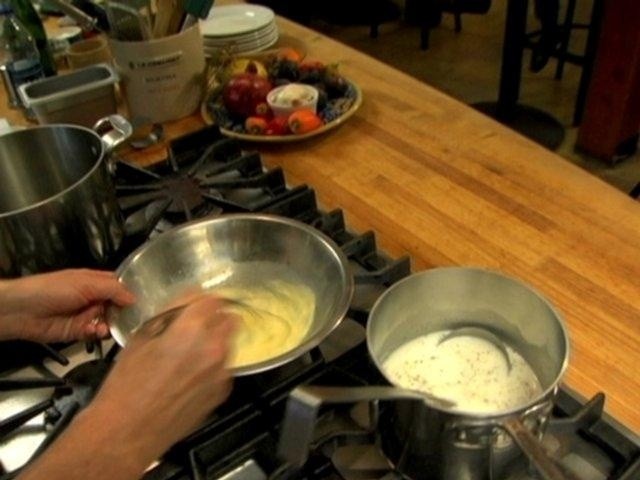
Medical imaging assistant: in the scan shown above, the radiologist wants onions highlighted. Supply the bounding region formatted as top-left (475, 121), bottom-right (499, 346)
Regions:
top-left (223, 63), bottom-right (272, 119)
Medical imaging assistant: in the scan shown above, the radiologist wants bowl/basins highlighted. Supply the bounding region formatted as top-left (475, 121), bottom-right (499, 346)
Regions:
top-left (107, 213), bottom-right (356, 380)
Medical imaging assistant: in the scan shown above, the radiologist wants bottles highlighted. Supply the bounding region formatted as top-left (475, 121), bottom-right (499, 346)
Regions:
top-left (0, 6), bottom-right (46, 111)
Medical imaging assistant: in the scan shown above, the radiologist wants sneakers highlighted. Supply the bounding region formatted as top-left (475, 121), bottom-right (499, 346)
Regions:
top-left (529, 24), bottom-right (562, 73)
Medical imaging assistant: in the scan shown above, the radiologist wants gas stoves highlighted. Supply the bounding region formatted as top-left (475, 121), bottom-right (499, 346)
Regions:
top-left (0, 123), bottom-right (640, 479)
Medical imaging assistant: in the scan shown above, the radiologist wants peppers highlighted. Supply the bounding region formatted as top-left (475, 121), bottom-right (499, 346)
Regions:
top-left (245, 111), bottom-right (322, 135)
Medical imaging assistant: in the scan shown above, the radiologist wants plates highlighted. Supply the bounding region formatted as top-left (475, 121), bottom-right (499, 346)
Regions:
top-left (197, 4), bottom-right (363, 143)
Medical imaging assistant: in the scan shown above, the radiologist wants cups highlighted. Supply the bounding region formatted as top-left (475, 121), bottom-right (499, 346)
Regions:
top-left (66, 38), bottom-right (125, 106)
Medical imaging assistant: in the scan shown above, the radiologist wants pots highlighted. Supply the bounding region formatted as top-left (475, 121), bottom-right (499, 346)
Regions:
top-left (0, 114), bottom-right (134, 275)
top-left (366, 266), bottom-right (571, 479)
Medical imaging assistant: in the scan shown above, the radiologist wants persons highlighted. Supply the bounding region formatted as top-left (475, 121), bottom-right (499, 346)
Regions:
top-left (0, 267), bottom-right (245, 480)
